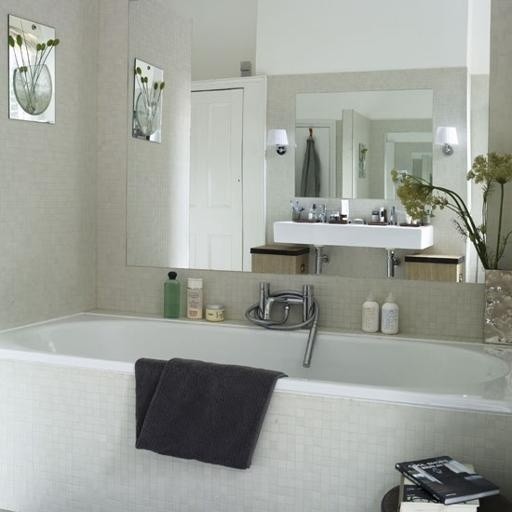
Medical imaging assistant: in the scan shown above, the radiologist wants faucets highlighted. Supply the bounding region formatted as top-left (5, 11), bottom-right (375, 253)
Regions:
top-left (330, 211), bottom-right (348, 223)
top-left (264, 296), bottom-right (290, 320)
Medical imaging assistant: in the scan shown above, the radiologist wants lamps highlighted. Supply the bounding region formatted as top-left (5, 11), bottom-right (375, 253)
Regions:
top-left (433, 126), bottom-right (460, 156)
top-left (266, 127), bottom-right (289, 156)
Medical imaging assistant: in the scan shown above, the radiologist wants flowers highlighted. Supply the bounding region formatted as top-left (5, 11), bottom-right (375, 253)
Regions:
top-left (390, 152), bottom-right (512, 270)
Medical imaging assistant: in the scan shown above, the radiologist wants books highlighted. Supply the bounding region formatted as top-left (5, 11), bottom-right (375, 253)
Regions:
top-left (395, 455), bottom-right (500, 512)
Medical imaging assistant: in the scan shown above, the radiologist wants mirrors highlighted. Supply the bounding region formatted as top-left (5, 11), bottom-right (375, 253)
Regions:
top-left (294, 90), bottom-right (434, 201)
top-left (124, 0), bottom-right (512, 286)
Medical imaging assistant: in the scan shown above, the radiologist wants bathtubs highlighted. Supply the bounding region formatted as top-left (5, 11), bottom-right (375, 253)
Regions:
top-left (0, 311), bottom-right (512, 416)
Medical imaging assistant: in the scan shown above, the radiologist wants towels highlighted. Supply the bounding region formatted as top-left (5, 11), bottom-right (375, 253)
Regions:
top-left (134, 358), bottom-right (290, 470)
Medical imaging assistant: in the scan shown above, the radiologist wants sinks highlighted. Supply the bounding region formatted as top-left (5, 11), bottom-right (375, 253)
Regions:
top-left (274, 221), bottom-right (434, 250)
